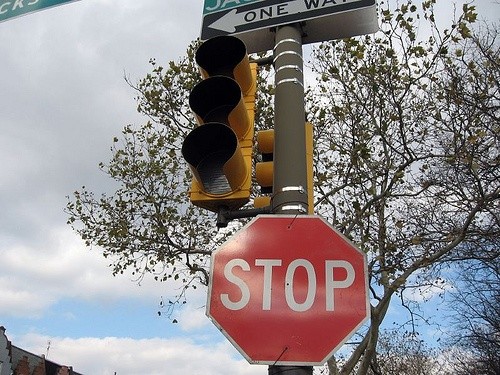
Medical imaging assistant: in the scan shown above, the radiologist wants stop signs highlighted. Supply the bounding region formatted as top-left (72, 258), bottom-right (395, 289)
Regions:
top-left (207, 212), bottom-right (372, 368)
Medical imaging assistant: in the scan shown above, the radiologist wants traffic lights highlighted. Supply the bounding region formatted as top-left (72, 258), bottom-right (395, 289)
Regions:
top-left (181, 34), bottom-right (254, 199)
top-left (256, 121), bottom-right (315, 215)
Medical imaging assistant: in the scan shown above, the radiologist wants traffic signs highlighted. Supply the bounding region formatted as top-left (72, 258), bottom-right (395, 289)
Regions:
top-left (0, 1), bottom-right (81, 23)
top-left (201, 0), bottom-right (379, 54)
